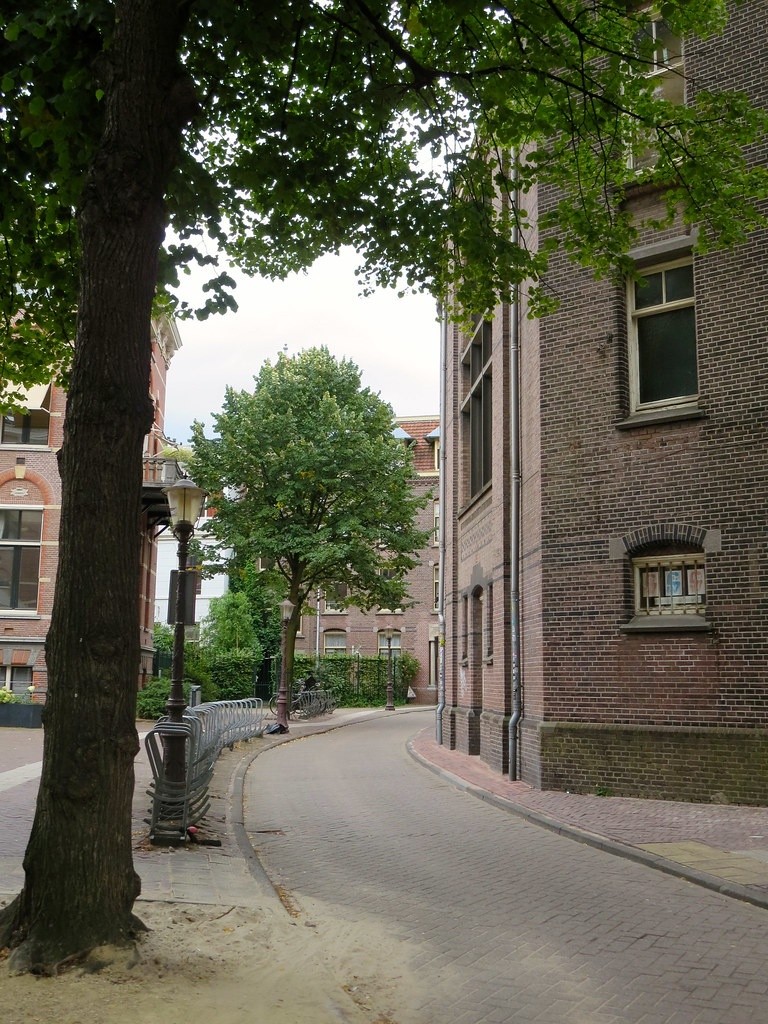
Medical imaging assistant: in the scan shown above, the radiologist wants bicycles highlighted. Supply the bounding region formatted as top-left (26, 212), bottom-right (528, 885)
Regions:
top-left (269, 682), bottom-right (337, 720)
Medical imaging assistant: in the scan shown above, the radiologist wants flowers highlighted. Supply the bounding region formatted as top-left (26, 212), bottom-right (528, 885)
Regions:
top-left (0, 686), bottom-right (38, 704)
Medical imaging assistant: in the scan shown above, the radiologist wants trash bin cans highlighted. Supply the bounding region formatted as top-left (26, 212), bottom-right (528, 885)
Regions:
top-left (189, 685), bottom-right (202, 708)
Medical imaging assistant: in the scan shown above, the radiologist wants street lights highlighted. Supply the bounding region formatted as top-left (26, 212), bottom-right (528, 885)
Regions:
top-left (275, 598), bottom-right (298, 733)
top-left (383, 624), bottom-right (396, 711)
top-left (161, 472), bottom-right (209, 822)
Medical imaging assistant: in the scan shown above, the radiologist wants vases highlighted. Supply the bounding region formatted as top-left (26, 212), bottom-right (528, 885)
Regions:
top-left (0, 703), bottom-right (42, 728)
